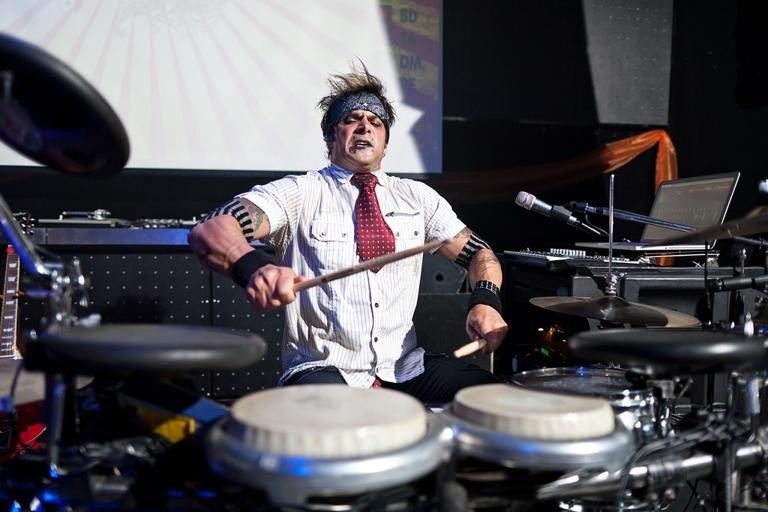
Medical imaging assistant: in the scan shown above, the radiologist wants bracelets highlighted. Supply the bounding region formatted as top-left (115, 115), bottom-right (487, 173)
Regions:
top-left (472, 280), bottom-right (501, 297)
top-left (463, 289), bottom-right (505, 315)
top-left (229, 246), bottom-right (278, 290)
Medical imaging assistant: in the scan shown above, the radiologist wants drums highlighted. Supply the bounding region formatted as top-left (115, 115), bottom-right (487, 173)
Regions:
top-left (207, 384), bottom-right (453, 512)
top-left (511, 366), bottom-right (658, 438)
top-left (439, 384), bottom-right (635, 512)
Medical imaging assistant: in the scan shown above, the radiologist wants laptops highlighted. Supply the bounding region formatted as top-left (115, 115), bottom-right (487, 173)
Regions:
top-left (574, 171), bottom-right (742, 251)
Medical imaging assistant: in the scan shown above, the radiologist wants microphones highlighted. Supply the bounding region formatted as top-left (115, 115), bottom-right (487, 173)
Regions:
top-left (514, 191), bottom-right (601, 238)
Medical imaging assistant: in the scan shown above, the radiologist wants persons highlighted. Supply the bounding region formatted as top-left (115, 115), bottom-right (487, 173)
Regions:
top-left (184, 57), bottom-right (512, 408)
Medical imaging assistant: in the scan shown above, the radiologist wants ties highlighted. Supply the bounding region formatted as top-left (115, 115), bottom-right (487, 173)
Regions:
top-left (352, 174), bottom-right (395, 274)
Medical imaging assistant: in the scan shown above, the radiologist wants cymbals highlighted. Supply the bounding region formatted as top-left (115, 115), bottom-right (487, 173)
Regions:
top-left (530, 296), bottom-right (703, 329)
top-left (642, 206), bottom-right (768, 246)
